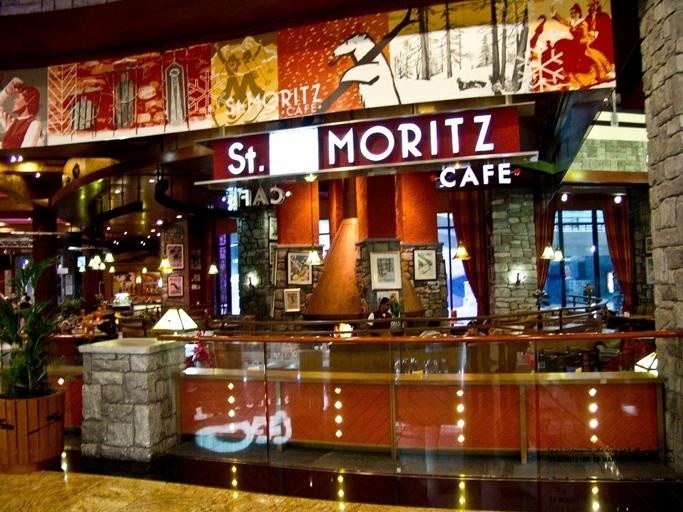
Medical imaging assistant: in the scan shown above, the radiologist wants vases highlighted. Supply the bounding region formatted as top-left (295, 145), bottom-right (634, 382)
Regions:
top-left (390, 318), bottom-right (406, 336)
top-left (535, 315), bottom-right (544, 332)
top-left (585, 306), bottom-right (596, 318)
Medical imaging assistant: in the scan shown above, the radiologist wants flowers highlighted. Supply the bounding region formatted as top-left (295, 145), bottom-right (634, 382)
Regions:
top-left (388, 292), bottom-right (403, 321)
top-left (584, 285), bottom-right (593, 307)
top-left (532, 289), bottom-right (549, 315)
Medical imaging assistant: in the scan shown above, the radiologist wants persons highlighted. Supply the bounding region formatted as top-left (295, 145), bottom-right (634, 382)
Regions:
top-left (0, 76), bottom-right (42, 150)
top-left (464, 321), bottom-right (487, 336)
top-left (21, 292), bottom-right (32, 304)
top-left (583, 283), bottom-right (596, 301)
top-left (420, 320), bottom-right (442, 337)
top-left (364, 297), bottom-right (394, 336)
top-left (20, 295), bottom-right (32, 309)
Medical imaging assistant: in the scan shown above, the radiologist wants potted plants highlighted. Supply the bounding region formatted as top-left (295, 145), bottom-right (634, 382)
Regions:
top-left (0, 251), bottom-right (65, 464)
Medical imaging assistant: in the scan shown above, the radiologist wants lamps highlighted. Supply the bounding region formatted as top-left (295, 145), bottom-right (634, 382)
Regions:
top-left (153, 307), bottom-right (199, 336)
top-left (452, 241), bottom-right (471, 260)
top-left (558, 184), bottom-right (575, 203)
top-left (157, 226), bottom-right (173, 272)
top-left (539, 240), bottom-right (564, 261)
top-left (609, 186), bottom-right (628, 205)
top-left (78, 253), bottom-right (117, 273)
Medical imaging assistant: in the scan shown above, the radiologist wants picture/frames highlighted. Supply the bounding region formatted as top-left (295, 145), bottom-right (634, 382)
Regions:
top-left (283, 288), bottom-right (301, 312)
top-left (165, 243), bottom-right (185, 271)
top-left (645, 256), bottom-right (655, 284)
top-left (287, 251), bottom-right (313, 286)
top-left (369, 251), bottom-right (402, 291)
top-left (643, 235), bottom-right (652, 257)
top-left (268, 216), bottom-right (278, 242)
top-left (168, 275), bottom-right (185, 298)
top-left (413, 246), bottom-right (437, 281)
